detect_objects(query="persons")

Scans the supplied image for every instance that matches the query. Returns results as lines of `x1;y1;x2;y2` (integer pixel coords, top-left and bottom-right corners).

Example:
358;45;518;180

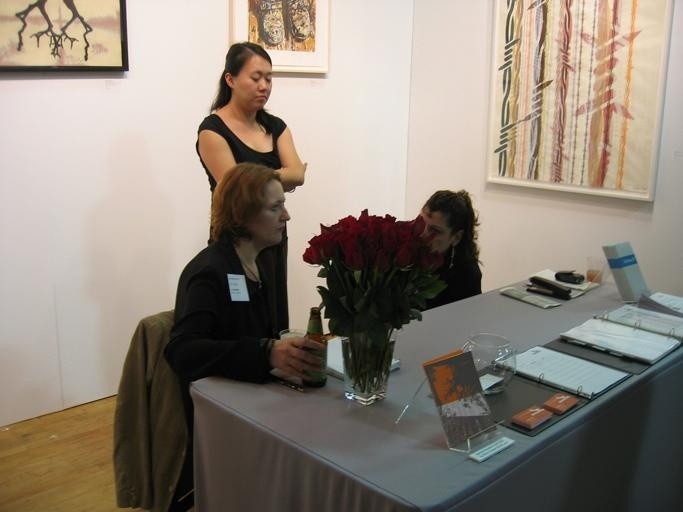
196;41;306;334
416;188;481;309
171;165;325;386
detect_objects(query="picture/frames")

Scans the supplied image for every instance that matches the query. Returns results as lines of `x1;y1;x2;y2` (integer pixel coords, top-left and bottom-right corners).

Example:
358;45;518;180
229;0;329;75
0;0;127;71
486;0;674;202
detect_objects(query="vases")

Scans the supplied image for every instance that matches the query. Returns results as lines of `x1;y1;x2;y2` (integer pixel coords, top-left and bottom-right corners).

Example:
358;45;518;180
342;337;396;405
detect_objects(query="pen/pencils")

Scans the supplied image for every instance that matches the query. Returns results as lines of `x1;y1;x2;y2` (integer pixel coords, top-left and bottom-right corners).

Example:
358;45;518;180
270;374;304;392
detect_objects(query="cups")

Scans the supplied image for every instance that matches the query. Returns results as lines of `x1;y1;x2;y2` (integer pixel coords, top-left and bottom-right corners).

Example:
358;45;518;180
585;255;606;283
278;329;302;339
461;332;516;395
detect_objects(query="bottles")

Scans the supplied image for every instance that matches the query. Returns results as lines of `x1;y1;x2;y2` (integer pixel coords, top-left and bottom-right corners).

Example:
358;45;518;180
301;307;328;388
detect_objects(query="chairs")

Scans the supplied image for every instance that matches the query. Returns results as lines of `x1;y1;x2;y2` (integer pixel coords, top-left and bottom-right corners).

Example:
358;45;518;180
114;310;225;512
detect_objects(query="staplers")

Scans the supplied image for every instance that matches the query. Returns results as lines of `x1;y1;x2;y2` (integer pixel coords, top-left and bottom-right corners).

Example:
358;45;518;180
525;276;572;301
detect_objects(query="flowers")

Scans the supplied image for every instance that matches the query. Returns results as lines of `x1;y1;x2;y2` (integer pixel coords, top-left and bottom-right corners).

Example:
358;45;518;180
302;209;448;390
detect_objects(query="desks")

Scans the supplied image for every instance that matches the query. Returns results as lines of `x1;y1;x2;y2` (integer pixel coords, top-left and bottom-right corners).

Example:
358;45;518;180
188;265;683;511
